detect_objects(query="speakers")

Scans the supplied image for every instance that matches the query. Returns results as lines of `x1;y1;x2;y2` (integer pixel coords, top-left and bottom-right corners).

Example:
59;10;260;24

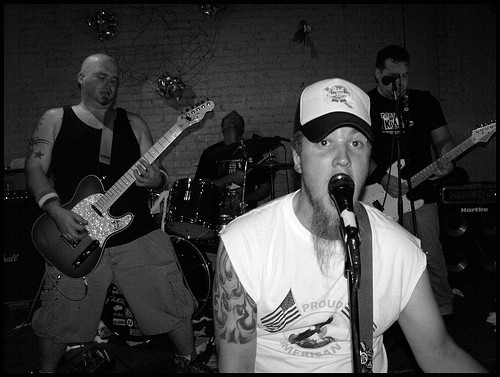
435;185;499;278
1;200;52;309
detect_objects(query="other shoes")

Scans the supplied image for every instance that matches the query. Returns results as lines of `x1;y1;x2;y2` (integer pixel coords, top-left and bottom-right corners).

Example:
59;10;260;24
169;354;211;374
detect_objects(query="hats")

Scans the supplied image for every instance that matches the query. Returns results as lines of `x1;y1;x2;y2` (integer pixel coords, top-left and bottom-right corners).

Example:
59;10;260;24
294;77;375;145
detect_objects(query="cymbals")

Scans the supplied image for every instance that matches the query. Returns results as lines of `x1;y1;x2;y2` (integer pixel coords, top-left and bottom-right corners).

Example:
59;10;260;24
227;135;281;156
249;161;294;170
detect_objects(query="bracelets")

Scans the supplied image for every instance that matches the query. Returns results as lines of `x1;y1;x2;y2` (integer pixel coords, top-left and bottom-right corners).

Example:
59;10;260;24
154;169;170;193
38;192;59;209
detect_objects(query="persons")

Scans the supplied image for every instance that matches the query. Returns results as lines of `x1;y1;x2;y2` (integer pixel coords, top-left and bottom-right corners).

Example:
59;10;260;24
364;43;457;374
212;77;492;374
193;110;277;211
24;53;217;377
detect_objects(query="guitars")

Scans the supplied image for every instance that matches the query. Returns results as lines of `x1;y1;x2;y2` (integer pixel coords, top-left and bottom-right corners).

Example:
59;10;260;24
30;97;216;279
358;119;498;224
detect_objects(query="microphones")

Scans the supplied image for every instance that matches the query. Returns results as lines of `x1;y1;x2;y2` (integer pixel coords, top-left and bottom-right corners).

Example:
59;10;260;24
382;74;399;86
329;172;362;270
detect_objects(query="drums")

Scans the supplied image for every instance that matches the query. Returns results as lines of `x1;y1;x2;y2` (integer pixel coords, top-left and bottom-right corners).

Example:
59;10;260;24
99;235;214;344
166;175;225;242
